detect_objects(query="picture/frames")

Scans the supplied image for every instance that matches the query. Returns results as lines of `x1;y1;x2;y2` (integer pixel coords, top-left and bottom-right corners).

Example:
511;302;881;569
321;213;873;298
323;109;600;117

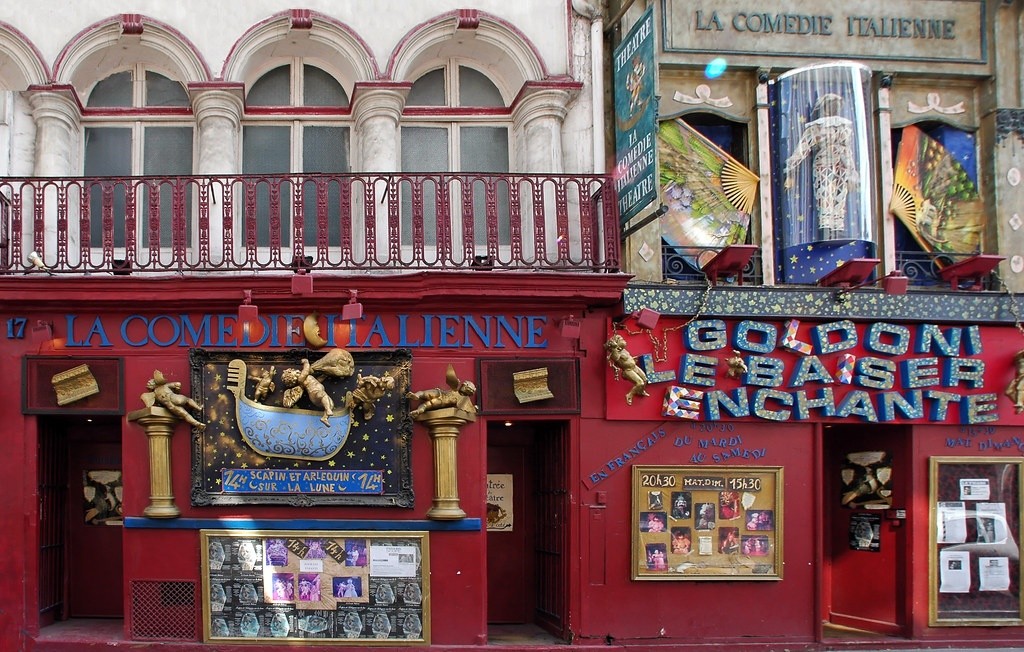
199;529;432;648
631;464;785;581
928;456;1024;626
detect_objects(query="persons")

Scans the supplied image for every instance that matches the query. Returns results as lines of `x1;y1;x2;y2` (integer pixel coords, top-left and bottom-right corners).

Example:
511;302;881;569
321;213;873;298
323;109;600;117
603;333;651;406
854;521;874;539
783;93;862;230
138;369;209;430
1004;349;1024;414
206;536;423;639
645;490;774;570
405;363;477;418
248;347;396;429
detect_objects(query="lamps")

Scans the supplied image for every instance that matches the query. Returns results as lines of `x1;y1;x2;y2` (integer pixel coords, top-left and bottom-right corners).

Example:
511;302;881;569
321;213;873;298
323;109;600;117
558;314;582;338
817;253;1007;304
32;319;53;342
471;255;494;271
613;308;661;330
27;251;55;277
238;256;363;321
112;259;132;276
701;243;759;287
